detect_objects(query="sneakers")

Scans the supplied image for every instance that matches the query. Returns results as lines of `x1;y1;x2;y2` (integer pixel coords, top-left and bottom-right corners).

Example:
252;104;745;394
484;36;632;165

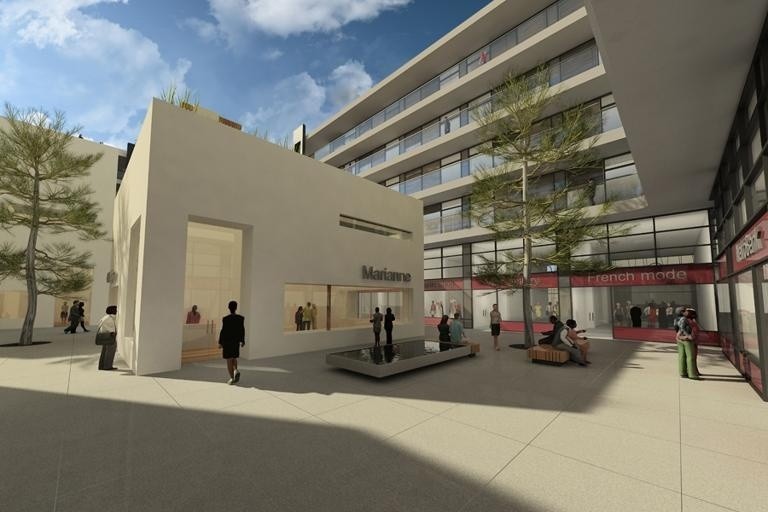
234;369;240;382
227;377;234;385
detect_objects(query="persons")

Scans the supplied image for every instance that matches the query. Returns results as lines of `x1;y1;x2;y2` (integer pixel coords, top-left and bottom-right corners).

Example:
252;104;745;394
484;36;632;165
613;300;706;381
429;300;436;317
294;302;318;331
383;307;395;344
489;304;502;351
449;313;470;343
437;314;451;342
218;301;246;384
98;306;119;371
60;300;91;334
370;307;383;346
186;305;200;324
533;300;592;367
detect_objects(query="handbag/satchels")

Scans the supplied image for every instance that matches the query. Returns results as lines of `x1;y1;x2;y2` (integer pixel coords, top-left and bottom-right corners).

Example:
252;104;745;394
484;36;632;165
95;332;115;345
